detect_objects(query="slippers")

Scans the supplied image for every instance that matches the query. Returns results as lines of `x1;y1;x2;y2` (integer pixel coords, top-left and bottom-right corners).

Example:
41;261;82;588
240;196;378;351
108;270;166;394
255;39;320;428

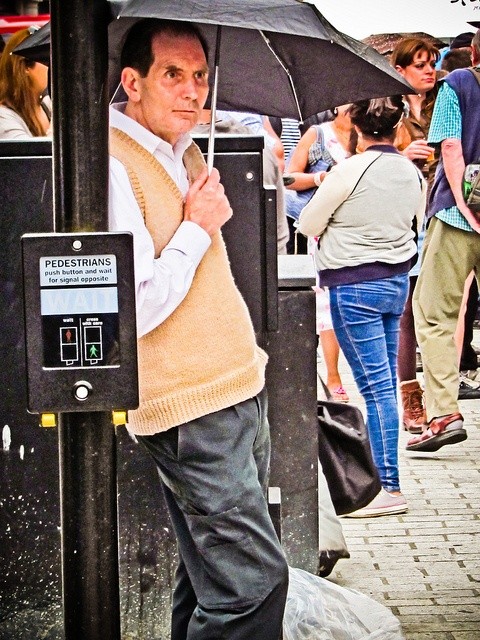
331;387;350;405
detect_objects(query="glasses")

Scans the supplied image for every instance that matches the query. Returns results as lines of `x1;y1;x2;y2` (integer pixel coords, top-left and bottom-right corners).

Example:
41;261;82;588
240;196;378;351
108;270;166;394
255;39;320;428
28;24;40;35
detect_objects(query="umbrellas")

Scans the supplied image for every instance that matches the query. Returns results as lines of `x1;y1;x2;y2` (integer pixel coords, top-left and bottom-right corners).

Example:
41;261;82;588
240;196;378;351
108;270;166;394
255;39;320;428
8;0;422;179
360;31;449;63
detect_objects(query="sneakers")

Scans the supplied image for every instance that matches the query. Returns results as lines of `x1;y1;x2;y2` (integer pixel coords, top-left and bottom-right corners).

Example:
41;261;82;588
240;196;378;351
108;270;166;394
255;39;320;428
341;486;407;518
458;382;479;399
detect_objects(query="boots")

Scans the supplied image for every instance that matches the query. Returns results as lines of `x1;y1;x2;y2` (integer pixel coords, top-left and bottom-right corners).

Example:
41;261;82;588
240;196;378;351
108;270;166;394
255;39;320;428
401;379;427;434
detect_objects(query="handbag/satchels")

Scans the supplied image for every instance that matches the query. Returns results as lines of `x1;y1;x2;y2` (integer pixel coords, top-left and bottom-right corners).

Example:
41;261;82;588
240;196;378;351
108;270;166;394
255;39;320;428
284;161;331;221
317;399;383;517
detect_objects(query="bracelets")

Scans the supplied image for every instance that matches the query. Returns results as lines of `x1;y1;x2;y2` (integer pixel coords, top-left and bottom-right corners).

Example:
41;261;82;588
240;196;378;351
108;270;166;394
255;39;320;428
313;171;325;186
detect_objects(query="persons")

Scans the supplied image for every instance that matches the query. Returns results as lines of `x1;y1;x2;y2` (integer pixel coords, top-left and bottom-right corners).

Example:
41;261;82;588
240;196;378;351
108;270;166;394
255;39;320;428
450;32;476;48
189;108;290;252
0;26;54;141
405;27;480;452
298;93;427;519
391;38;448;434
105;18;288;640
457;269;480;400
441;47;473;73
456;276;480;383
436;70;451;81
282;100;364;405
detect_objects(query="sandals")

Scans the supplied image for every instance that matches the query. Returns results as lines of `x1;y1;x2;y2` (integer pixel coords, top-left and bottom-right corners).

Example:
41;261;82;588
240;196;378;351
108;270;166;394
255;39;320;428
406;414;470;452
